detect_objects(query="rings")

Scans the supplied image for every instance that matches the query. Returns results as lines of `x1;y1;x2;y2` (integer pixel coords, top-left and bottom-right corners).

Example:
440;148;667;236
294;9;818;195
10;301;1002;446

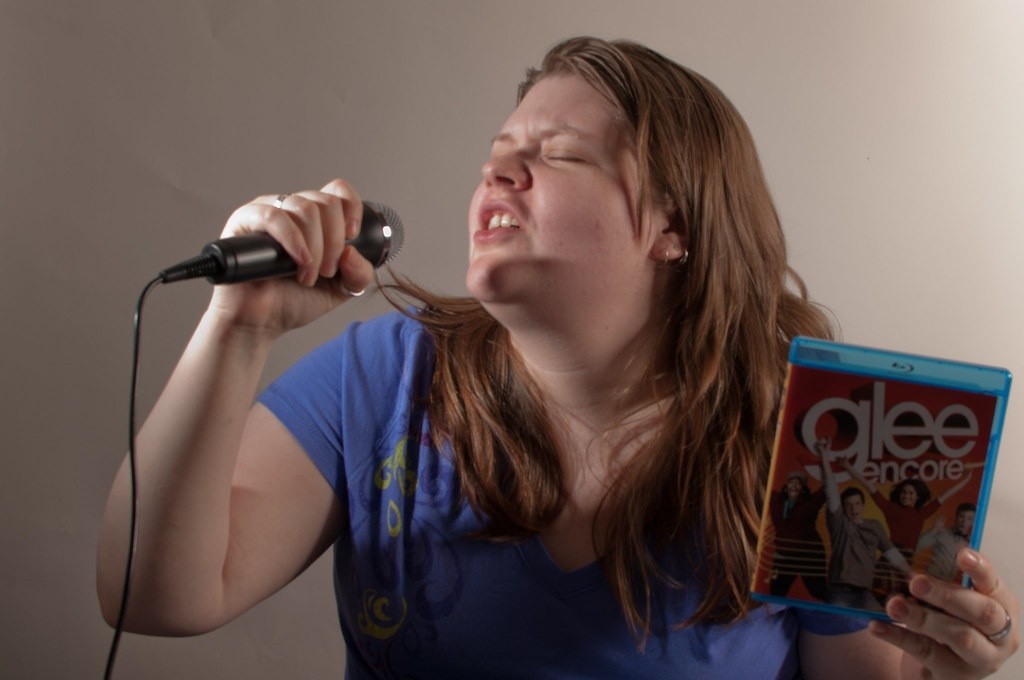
274;192;292;208
339;282;365;297
988;610;1013;640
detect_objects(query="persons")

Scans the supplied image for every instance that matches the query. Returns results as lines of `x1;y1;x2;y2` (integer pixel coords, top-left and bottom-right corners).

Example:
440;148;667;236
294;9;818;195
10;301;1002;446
835;453;974;608
814;435;914;612
766;475;831;601
915;500;976;584
94;35;1023;680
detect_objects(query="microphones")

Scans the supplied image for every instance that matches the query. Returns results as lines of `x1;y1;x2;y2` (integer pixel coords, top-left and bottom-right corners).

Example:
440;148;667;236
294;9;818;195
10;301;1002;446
159;201;406;289
